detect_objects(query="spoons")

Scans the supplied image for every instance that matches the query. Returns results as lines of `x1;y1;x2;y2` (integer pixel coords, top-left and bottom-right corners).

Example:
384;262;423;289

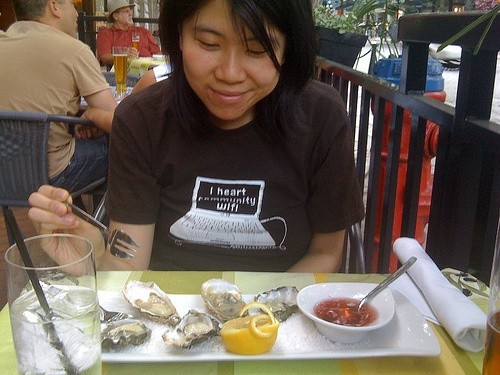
352;257;417;326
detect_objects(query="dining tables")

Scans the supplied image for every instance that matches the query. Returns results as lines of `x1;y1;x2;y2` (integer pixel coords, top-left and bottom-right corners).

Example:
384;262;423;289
0;272;489;375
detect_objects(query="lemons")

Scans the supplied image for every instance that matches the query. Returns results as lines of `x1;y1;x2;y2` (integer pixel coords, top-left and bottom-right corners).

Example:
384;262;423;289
220;302;280;354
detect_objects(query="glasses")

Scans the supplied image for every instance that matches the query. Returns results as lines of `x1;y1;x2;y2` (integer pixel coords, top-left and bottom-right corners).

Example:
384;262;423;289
116;8;133;14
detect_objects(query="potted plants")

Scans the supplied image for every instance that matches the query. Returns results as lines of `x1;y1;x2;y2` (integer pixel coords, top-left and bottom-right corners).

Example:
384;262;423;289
312;0;404;68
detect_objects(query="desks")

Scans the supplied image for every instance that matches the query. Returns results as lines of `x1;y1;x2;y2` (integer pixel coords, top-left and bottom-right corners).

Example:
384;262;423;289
78;71;137;111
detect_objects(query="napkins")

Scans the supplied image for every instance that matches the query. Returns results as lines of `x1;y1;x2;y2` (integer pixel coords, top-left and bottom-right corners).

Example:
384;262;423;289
388;237;487;351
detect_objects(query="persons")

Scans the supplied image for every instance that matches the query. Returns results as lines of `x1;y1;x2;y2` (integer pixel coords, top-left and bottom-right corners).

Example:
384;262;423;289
96;0;160;68
74;64;173;140
0;0;117;229
28;0;365;274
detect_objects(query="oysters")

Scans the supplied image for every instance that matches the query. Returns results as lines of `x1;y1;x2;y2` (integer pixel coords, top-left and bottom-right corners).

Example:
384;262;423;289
162;308;223;351
122;279;182;327
200;278;249;324
251;284;299;324
100;318;153;352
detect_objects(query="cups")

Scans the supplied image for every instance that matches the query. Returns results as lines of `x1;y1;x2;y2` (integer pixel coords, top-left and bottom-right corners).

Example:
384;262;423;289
131;32;140;59
4;234;103;375
112;45;129;104
480;220;500;374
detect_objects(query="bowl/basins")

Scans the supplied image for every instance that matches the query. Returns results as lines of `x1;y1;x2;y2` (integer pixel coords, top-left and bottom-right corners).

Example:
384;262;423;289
296;281;395;346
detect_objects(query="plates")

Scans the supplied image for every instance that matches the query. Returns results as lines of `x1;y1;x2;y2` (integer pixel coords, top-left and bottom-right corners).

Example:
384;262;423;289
98;289;441;362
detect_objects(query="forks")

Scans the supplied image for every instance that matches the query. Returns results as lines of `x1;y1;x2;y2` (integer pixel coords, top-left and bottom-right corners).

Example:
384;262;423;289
65;200;141;260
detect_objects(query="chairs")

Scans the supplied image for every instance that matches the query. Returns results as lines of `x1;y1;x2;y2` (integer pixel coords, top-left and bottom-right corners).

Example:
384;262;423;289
0;112;367;273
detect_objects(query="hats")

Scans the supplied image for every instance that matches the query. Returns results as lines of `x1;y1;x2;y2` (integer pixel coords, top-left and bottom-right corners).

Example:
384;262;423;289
107;0;135;23
